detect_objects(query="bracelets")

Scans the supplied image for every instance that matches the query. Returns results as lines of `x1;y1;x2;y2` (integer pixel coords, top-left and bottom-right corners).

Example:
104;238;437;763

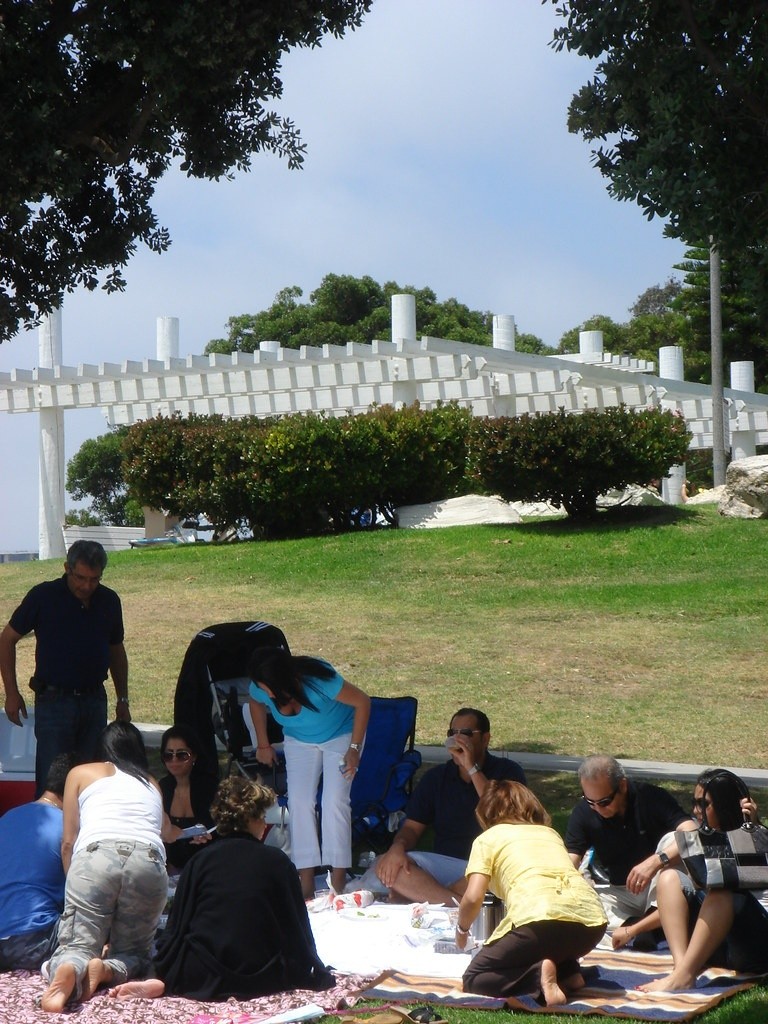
625;925;634;939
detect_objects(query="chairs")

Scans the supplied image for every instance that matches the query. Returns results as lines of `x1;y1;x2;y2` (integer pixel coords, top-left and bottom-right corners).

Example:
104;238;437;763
277;695;422;880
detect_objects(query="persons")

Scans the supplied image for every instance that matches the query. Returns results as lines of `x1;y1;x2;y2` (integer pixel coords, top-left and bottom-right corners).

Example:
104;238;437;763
1;619;768;1014
0;540;129;800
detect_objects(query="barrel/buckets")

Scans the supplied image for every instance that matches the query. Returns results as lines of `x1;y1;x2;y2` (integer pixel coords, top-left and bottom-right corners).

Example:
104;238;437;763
472;893;506;941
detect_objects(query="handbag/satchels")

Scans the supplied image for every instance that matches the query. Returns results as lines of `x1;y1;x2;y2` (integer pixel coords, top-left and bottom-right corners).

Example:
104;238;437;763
674;774;768;891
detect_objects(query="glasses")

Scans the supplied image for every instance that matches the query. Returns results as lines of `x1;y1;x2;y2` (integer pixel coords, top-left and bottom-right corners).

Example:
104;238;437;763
447;727;482;737
68;567;102;583
692;797;713;808
584;784;618;807
162;751;194;762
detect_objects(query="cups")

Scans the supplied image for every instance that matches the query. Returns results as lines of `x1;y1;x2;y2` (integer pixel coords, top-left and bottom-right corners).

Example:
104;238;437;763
315;888;333;912
444;909;459;932
445;735;461;751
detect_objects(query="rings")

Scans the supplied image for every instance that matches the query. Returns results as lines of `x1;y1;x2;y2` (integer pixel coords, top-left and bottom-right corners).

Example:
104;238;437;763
637;881;642;886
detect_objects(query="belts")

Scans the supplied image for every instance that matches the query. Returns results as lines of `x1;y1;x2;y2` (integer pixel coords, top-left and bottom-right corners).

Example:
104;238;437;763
45;685;83;695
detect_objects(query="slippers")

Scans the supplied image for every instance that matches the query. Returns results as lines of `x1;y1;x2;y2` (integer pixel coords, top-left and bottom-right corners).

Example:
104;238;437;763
341;1013;404;1024
389;1003;449;1024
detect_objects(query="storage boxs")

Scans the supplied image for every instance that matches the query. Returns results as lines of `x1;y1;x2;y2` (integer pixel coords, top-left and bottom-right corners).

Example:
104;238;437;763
0;707;39;816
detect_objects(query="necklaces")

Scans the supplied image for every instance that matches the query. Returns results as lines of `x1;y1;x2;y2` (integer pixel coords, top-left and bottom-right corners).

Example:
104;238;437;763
41;796;63;811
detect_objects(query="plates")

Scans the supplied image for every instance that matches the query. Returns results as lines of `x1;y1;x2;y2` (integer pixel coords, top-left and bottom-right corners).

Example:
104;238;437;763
338;908;390;922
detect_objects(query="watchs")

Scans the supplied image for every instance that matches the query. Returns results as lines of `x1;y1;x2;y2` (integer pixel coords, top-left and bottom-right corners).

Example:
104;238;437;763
467;763;481;775
348;743;362;753
117;698;129;705
657;850;670;869
456;923;469;935
